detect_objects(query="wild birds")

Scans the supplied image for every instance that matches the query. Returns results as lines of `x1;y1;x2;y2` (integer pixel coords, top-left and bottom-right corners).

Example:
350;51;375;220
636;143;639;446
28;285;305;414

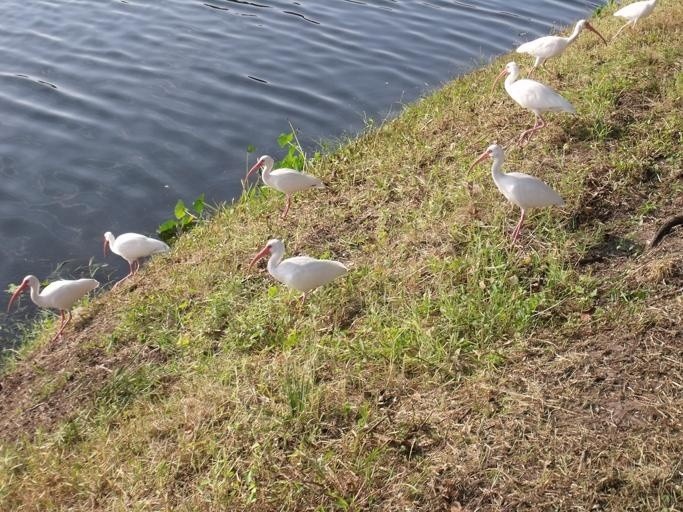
612;0;658;39
467;144;567;253
492;61;577;146
516;19;607;79
249;239;350;308
246;155;326;218
103;232;171;291
7;275;100;342
